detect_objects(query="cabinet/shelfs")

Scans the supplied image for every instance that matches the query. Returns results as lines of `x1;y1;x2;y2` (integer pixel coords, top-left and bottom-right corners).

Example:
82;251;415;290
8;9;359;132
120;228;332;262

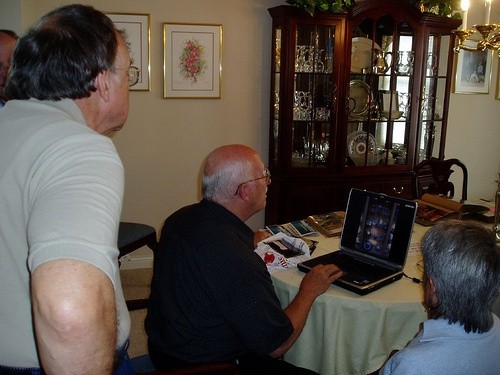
265;0;462;229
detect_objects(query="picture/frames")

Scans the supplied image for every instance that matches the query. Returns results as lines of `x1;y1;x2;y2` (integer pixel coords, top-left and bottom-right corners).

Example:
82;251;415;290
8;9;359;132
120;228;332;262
161;22;222;99
100;12;151;92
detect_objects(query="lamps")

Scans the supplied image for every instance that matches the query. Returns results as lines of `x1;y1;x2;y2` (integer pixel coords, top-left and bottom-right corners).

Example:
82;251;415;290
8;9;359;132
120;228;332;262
453;0;500;57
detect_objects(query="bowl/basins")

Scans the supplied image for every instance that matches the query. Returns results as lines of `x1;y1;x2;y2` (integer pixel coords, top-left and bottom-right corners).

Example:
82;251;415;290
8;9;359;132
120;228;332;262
378;149;405;164
382;111;404;119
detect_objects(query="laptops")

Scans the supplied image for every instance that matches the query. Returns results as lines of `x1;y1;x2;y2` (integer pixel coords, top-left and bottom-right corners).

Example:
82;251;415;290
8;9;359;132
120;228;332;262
296;187;418;296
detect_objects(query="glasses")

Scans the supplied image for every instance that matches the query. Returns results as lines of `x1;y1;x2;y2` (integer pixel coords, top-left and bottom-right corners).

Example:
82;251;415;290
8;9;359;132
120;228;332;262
417;259;425;272
234;168;271;195
109;65;140;86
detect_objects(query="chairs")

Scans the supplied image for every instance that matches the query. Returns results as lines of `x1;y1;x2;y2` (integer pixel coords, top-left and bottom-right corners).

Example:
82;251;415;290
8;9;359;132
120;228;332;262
412;157;468;201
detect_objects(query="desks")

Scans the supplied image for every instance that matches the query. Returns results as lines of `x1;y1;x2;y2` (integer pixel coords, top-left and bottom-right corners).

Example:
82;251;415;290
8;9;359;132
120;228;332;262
269;201;500;375
453;37;492;95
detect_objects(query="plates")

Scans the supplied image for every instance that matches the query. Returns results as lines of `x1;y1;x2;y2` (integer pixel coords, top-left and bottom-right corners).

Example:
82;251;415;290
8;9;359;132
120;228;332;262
349;80;373;116
350;37;381;73
348;131;377;163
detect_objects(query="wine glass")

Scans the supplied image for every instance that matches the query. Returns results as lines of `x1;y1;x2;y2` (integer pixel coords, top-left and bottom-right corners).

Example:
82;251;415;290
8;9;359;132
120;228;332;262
295;44;325;72
274;90;330;120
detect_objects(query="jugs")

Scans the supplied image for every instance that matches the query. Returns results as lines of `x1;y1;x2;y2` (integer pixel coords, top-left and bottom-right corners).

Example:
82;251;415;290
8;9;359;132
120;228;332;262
370;48;438;76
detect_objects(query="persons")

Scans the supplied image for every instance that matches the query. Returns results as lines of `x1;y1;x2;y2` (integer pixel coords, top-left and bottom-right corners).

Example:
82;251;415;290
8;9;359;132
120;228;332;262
463;59;484;83
378;219;500;375
0;3;141;375
143;145;344;375
